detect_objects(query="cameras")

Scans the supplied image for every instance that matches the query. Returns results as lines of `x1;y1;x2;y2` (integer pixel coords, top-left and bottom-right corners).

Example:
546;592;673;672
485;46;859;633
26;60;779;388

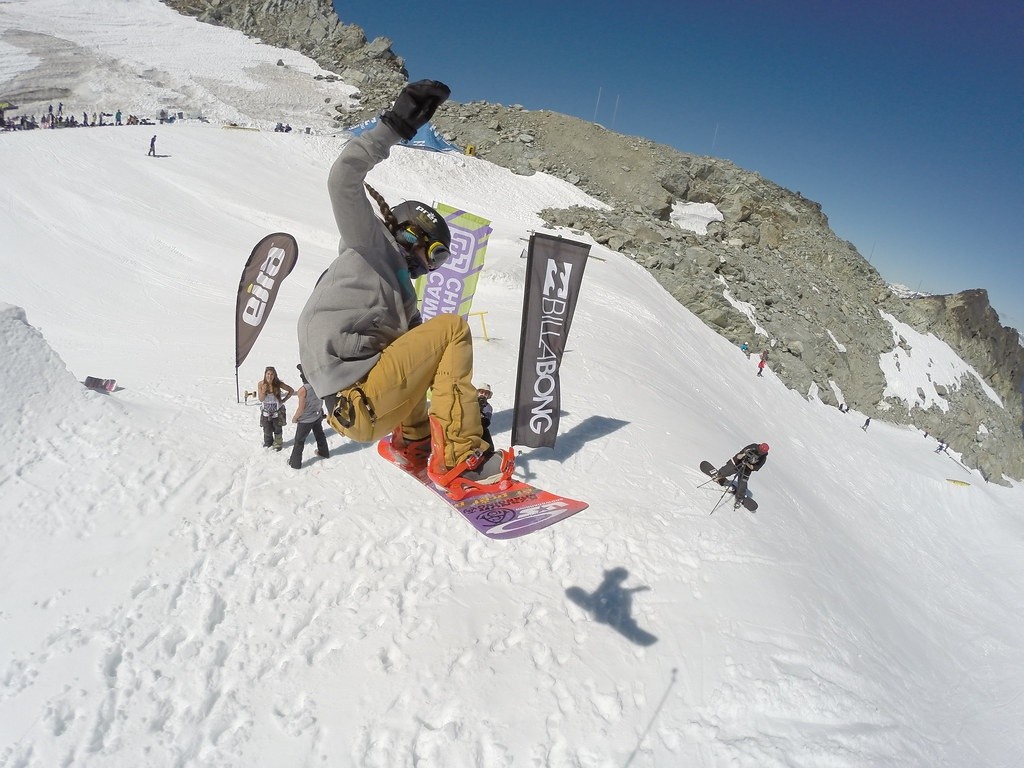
477;394;488;407
744;455;751;461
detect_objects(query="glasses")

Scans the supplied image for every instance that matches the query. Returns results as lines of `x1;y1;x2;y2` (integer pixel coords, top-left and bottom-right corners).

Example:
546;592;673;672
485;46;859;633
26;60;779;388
266;366;275;370
410;225;451;271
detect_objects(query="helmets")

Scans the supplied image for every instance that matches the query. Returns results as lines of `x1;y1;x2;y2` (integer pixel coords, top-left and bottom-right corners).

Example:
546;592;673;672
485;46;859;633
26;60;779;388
758;442;769;454
389;199;451;271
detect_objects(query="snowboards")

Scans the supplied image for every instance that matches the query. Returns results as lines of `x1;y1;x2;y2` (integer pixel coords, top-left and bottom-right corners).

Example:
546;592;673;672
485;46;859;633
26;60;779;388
699;459;759;512
82;375;118;392
376;434;590;542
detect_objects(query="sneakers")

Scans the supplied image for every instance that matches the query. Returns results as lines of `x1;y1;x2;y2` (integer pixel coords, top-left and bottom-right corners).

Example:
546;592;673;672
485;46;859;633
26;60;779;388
287;459;301;469
394;436;431;464
314;448;329;457
433;450;515;493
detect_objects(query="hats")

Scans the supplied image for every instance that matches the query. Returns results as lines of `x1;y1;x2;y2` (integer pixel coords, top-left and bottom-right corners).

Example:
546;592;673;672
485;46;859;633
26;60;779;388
745;342;748;345
297;364;303;372
477;382;493;400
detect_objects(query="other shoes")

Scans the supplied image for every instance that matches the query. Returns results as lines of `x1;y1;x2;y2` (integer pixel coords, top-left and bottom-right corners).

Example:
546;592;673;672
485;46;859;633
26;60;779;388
734;500;743;508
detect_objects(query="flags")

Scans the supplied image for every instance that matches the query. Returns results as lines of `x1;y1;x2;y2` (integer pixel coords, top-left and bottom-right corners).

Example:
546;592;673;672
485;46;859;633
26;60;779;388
510;233;592;448
414;202;493;324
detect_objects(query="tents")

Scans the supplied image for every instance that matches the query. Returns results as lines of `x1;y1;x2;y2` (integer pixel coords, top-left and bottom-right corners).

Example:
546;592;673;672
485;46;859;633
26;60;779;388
346;114;453;153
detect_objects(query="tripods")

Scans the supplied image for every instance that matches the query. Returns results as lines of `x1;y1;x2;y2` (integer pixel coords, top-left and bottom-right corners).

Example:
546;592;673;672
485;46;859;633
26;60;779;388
697;461;748;516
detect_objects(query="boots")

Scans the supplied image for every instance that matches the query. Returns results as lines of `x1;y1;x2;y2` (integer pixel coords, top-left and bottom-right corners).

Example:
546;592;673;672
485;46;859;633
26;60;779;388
263;432;274;449
274;434;283;452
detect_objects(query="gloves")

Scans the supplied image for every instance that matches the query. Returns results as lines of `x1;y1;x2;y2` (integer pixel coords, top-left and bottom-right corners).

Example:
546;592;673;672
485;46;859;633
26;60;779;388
382;78;451;140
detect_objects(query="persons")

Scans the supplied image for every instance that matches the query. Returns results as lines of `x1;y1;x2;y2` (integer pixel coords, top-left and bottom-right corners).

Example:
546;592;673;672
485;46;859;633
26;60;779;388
198;116;211;123
296;78;516;492
756;349;770;378
861;417;872;432
275;122;292;133
148;135;158;157
935;442;950;453
475;384;495;458
257;367;294;452
712;443;769;512
985;473;992;483
0;102;176;131
839;403;851;414
287;363;330;469
924;428;931;438
741;341;751;360
